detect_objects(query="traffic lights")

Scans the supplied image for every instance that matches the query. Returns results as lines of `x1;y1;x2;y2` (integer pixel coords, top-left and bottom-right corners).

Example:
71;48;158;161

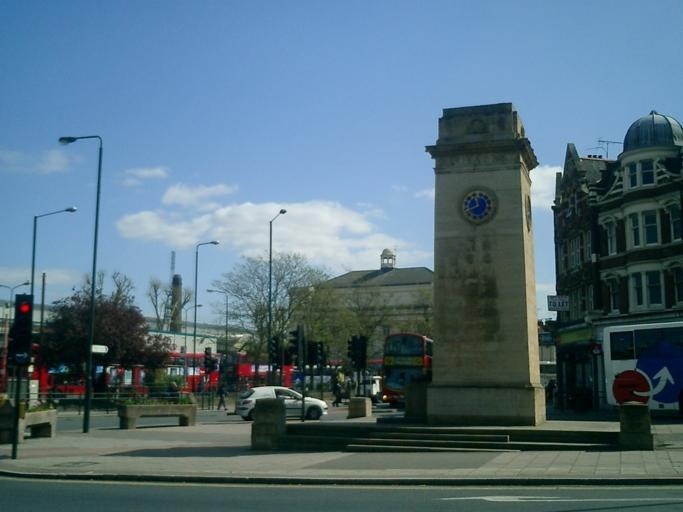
346;334;359;361
268;334;283;369
290;322;306;367
11;292;33;367
313;341;324;363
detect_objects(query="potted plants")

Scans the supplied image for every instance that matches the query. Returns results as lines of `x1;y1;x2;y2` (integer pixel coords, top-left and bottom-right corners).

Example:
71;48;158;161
0;399;57;443
118;396;198;429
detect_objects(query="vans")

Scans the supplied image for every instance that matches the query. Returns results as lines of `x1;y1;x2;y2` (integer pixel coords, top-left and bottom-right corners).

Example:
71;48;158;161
353;375;383;403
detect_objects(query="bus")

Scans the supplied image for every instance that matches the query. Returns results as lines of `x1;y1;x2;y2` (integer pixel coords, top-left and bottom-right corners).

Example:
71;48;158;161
288;363;363;391
2;347;297;395
600;319;682;420
539;360;557;393
325;359;383;377
378;331;433;410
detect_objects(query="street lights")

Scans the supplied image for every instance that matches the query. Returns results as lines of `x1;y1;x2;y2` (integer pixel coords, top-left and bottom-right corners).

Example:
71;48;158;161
29;203;81;301
189;238;224;393
54;132;103;432
205;286;230;363
182;304;205;389
1;277;32;383
266;205;287;384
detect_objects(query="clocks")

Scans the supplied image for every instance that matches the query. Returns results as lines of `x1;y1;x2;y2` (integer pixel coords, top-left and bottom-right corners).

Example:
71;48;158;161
525;195;531;231
459;186;498;226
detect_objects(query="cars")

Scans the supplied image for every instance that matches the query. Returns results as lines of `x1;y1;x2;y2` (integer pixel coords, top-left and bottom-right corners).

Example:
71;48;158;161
232;383;330;421
